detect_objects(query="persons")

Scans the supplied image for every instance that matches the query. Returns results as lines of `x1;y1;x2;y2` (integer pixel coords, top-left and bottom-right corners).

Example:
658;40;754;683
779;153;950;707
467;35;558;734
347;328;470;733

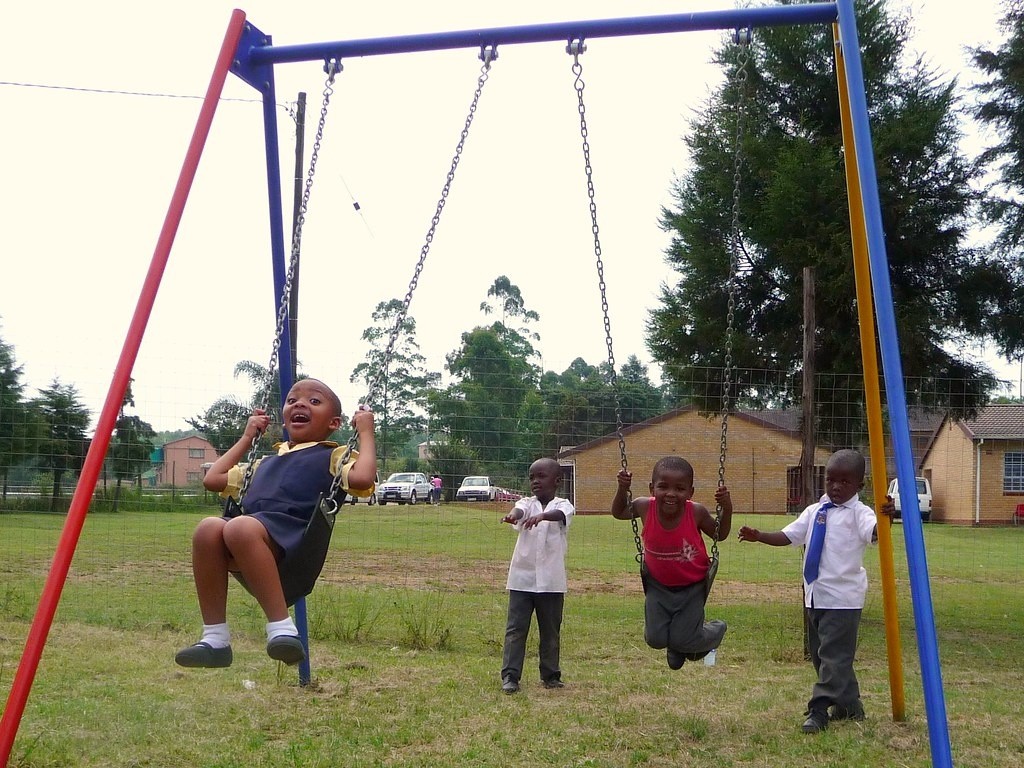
430;475;441;504
611;455;733;671
738;449;896;734
499;458;575;695
175;378;377;668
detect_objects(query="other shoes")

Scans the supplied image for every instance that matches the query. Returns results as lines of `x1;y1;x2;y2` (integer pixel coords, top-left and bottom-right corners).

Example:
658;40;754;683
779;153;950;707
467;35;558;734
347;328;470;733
267;635;305;666
175;641;233;668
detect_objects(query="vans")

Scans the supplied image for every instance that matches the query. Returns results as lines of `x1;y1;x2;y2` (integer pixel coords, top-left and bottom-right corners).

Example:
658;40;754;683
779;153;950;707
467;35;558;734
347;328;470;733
888;476;933;521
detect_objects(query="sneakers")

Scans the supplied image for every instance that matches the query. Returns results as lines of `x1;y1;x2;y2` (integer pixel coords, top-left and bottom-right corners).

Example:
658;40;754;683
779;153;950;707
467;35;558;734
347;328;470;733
686;649;713;661
667;646;686;670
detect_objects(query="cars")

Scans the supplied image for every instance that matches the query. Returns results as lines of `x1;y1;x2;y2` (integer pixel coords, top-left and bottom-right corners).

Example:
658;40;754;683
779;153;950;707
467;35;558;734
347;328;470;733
494;487;522;502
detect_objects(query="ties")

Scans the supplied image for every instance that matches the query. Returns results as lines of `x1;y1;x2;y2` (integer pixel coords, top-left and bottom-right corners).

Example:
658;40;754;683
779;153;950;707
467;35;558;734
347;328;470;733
804;502;834;585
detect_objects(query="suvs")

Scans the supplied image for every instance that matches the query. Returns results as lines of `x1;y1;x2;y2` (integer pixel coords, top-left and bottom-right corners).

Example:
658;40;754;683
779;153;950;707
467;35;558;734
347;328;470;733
455;476;495;502
341;471;380;506
376;472;435;505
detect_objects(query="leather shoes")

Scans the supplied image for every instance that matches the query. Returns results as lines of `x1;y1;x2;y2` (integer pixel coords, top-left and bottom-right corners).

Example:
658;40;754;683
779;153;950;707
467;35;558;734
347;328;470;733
801;711;828;731
503;674;519;694
542;679;564;689
828;707;865;720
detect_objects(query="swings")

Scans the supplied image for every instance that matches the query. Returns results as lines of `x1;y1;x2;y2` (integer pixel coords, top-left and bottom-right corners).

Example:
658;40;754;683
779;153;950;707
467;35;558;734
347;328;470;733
218;51;490;608
572;43;751;601
